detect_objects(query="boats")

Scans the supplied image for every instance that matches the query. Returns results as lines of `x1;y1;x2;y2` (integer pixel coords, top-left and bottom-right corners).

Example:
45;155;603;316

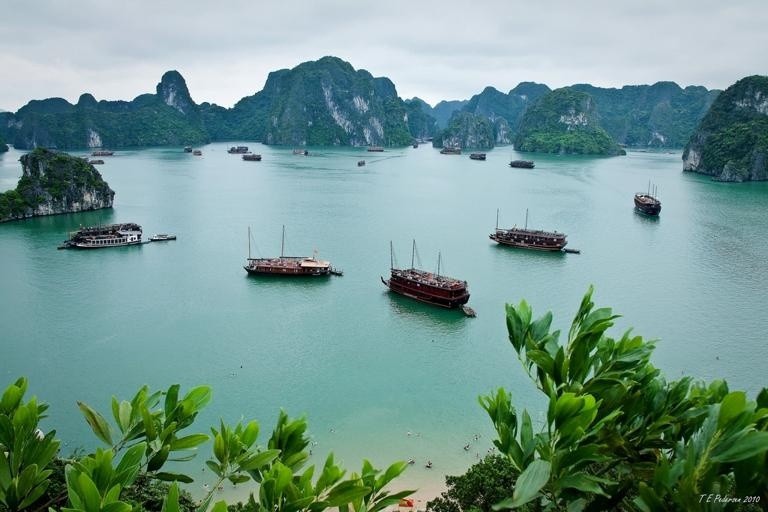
292;143;486;166
184;146;202;156
57;215;176;249
89;150;115;164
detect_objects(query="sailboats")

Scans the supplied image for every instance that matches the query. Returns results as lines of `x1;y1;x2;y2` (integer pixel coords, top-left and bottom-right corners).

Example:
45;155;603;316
488;208;580;254
243;225;343;277
381;239;478;318
226;143;261;161
507;153;535;168
633;179;661;216
